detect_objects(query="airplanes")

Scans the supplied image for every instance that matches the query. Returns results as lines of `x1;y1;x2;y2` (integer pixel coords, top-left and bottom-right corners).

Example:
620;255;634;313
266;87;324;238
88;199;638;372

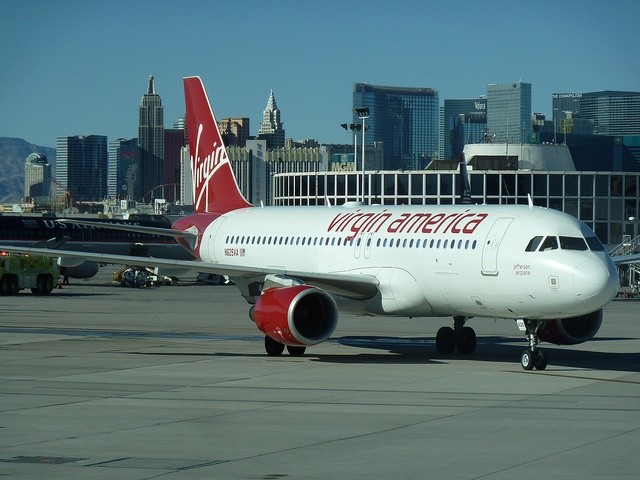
0;76;620;370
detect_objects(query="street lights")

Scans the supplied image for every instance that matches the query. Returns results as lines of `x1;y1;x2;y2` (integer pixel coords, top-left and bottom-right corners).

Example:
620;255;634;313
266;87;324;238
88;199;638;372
355;107;369;202
339;123;368;171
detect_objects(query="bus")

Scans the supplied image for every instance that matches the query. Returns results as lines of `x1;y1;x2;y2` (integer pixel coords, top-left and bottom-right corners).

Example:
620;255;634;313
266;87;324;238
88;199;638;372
1;251;59;297
